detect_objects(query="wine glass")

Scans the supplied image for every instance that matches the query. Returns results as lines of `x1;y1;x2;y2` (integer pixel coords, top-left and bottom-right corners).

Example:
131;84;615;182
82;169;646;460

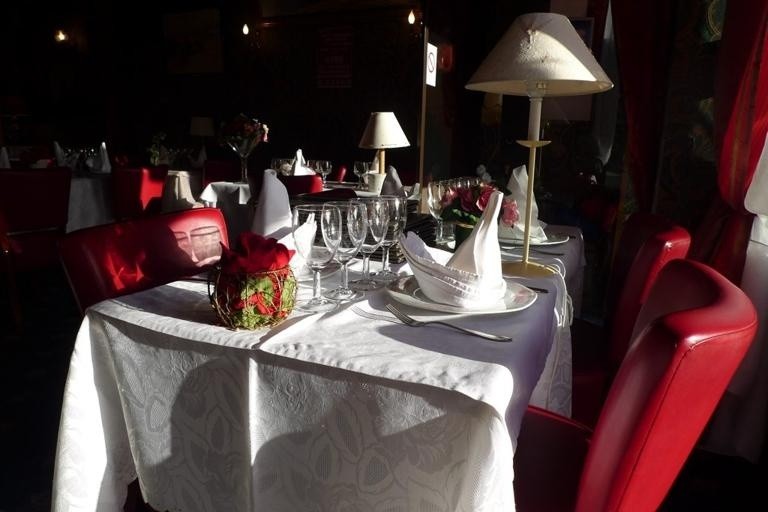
172;226;222;262
269;156;374;189
292;194;408;314
426;175;480;244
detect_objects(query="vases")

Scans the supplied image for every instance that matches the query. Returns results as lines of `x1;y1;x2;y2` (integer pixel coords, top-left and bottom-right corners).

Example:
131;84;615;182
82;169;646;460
454;221;480;251
211;269;297;331
223;134;261;182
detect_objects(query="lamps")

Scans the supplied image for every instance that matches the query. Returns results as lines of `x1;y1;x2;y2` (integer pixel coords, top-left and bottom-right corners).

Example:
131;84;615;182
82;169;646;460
464;12;615;279
357;111;412;172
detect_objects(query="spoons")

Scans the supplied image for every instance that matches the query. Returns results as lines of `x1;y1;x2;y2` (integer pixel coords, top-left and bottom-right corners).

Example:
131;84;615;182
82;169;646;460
385;302;513;342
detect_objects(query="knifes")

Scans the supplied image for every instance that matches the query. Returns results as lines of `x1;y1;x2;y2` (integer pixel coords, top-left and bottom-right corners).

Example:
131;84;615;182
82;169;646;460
499;246;566;257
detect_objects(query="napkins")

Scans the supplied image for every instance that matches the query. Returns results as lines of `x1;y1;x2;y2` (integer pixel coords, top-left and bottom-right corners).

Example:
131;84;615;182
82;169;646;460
380;166;406;198
400;189;504;310
494;166;548;244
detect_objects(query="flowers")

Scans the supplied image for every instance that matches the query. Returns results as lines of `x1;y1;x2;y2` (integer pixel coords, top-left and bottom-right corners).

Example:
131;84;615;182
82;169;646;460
438;181;519;228
220;111;271;143
208;229;297;330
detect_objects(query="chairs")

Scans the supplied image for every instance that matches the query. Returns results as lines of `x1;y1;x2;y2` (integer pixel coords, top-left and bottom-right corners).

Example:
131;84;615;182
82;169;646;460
572;226;691;421
513;259;758;510
1;147;360;318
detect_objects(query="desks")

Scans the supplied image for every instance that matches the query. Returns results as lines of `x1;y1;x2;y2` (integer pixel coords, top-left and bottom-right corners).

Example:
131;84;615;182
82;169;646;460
434;226;586;318
88;254;556;511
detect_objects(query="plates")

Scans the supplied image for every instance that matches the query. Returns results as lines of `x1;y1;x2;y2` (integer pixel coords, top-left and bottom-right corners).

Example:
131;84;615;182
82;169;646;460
497;229;570;245
386;277;537;317
